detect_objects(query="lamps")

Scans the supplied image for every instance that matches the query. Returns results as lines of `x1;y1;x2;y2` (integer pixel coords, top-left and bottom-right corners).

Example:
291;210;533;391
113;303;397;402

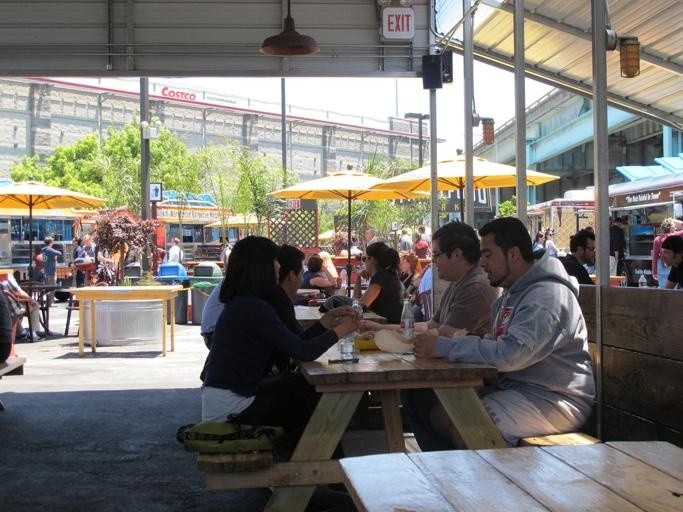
471;112;494;145
258;0;321;57
605;28;639;77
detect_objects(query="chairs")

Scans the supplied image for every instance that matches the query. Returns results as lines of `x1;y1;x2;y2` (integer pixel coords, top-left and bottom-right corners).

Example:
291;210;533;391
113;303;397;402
0;278;108;342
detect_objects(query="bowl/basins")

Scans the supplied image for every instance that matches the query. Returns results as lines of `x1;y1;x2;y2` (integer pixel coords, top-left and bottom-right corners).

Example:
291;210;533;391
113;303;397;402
587;275;625;286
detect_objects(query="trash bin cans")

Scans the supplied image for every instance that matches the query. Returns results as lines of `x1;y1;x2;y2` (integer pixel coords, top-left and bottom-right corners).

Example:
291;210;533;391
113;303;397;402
191;261;224;324
156;262;191;324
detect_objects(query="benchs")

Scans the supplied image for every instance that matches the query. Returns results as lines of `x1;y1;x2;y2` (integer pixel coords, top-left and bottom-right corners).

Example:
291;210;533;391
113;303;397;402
194;421;280;492
0;355;26;412
517;430;602;446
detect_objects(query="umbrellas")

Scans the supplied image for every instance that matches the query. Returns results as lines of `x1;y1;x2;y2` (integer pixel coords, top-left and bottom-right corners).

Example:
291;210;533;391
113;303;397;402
1;175;108;295
203;210;291;238
379;147;564;221
267;163;430;297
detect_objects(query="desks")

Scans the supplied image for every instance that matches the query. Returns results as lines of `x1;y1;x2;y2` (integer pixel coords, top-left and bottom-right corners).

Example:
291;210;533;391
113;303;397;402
250;302;509;511
337;440;683;512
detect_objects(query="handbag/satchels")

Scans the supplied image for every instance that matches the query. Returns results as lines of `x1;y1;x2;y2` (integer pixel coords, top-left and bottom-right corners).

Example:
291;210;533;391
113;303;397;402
319;295;370;313
34;253;45;263
174;413;284;455
617;259;640;286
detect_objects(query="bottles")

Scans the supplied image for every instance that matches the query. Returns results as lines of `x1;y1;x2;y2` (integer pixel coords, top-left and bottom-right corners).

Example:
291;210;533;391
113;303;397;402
620;271;627;287
351;300;364;319
339;334;355;364
637;275;647;288
400;298;415;338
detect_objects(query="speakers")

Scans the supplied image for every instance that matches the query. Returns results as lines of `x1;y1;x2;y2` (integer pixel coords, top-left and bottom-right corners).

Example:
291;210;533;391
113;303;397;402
441;50;454;85
423;53;442;87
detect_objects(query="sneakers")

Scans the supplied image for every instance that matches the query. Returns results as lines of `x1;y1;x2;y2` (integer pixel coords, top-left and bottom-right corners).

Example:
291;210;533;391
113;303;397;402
327;482;349;496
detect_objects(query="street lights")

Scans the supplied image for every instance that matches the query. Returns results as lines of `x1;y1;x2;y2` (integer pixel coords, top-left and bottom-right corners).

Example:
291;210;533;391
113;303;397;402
404;112;429;168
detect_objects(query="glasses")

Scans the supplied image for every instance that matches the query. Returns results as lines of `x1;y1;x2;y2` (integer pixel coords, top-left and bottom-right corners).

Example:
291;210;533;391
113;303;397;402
362;255;370;263
432;250;447;258
584;247;596;252
548;234;554;236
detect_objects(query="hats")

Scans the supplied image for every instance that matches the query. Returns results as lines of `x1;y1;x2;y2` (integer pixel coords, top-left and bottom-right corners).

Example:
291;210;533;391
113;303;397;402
661;235;683;251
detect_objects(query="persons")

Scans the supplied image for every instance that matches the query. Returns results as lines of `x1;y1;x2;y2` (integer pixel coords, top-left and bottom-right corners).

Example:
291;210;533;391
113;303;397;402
202;234;363;493
0;237;183;363
531;216;683;290
353;221;497;432
412;217;596;445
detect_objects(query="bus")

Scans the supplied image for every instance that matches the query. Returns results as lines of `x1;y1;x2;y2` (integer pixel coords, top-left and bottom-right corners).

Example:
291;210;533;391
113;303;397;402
0;209;104;270
